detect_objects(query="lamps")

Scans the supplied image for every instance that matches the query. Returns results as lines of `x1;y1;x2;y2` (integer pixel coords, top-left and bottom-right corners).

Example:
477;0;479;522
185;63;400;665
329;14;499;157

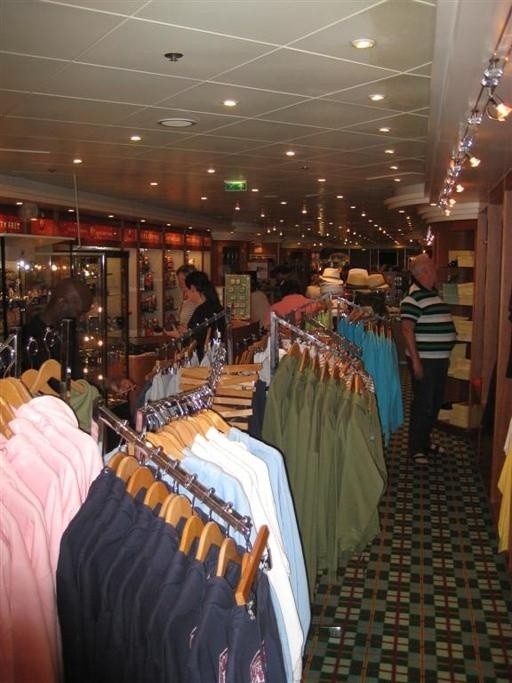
436;43;511;219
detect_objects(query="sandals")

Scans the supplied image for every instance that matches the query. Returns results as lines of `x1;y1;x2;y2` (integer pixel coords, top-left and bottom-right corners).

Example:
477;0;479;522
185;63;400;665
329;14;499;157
426;443;447;456
412;452;429;467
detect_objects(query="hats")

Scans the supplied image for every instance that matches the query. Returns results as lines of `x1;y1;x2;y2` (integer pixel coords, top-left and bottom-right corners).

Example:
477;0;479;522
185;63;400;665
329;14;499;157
319;267;390;293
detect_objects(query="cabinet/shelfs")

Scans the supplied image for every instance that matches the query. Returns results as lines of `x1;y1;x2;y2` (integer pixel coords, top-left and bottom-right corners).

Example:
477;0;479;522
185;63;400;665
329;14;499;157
0;198;248;449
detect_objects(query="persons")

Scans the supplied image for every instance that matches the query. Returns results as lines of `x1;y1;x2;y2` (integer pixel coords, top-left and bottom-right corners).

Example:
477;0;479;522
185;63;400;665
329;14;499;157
174;263;198;329
250;280;271;320
163;270;227;364
19;277;93;394
399;252;466;465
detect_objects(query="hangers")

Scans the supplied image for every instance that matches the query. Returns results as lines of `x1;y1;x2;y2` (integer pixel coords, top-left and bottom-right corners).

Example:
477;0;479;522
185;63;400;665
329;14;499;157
2;324;270;618
274;294;400;400
